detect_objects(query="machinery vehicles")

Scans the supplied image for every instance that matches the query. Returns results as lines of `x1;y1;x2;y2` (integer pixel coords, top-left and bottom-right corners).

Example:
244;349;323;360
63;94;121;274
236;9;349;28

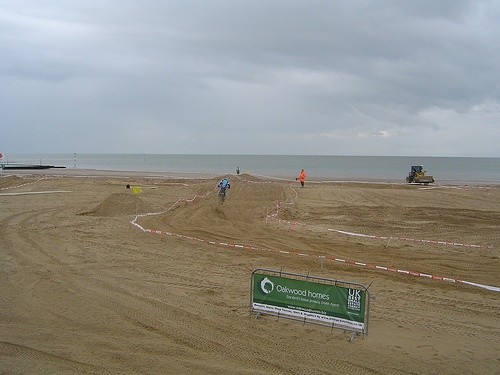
406;165;435;183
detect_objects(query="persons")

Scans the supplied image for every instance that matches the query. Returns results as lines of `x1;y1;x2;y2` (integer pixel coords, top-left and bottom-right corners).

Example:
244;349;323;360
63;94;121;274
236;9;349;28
216;177;231;203
297;168;306;188
235;166;241;175
